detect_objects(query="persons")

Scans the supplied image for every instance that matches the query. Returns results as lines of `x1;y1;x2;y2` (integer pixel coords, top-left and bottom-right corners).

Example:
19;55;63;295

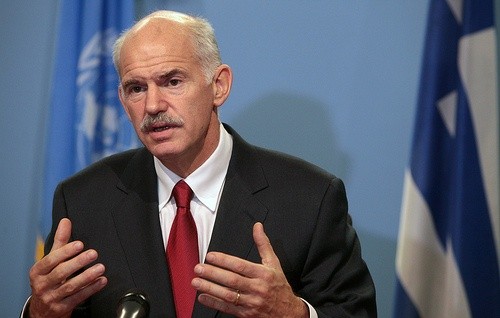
20;10;378;318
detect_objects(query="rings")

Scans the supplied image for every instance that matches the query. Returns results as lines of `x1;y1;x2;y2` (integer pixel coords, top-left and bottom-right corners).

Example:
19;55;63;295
235;290;240;306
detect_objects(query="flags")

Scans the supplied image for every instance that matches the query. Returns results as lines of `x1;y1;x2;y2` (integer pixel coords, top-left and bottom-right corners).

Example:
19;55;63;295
34;0;145;262
391;0;499;318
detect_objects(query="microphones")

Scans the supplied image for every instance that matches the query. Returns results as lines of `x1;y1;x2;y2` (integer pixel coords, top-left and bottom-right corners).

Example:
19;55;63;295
115;288;151;318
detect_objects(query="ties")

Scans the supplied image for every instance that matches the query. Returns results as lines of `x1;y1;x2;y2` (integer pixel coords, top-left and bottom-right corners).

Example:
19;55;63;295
165;180;199;318
